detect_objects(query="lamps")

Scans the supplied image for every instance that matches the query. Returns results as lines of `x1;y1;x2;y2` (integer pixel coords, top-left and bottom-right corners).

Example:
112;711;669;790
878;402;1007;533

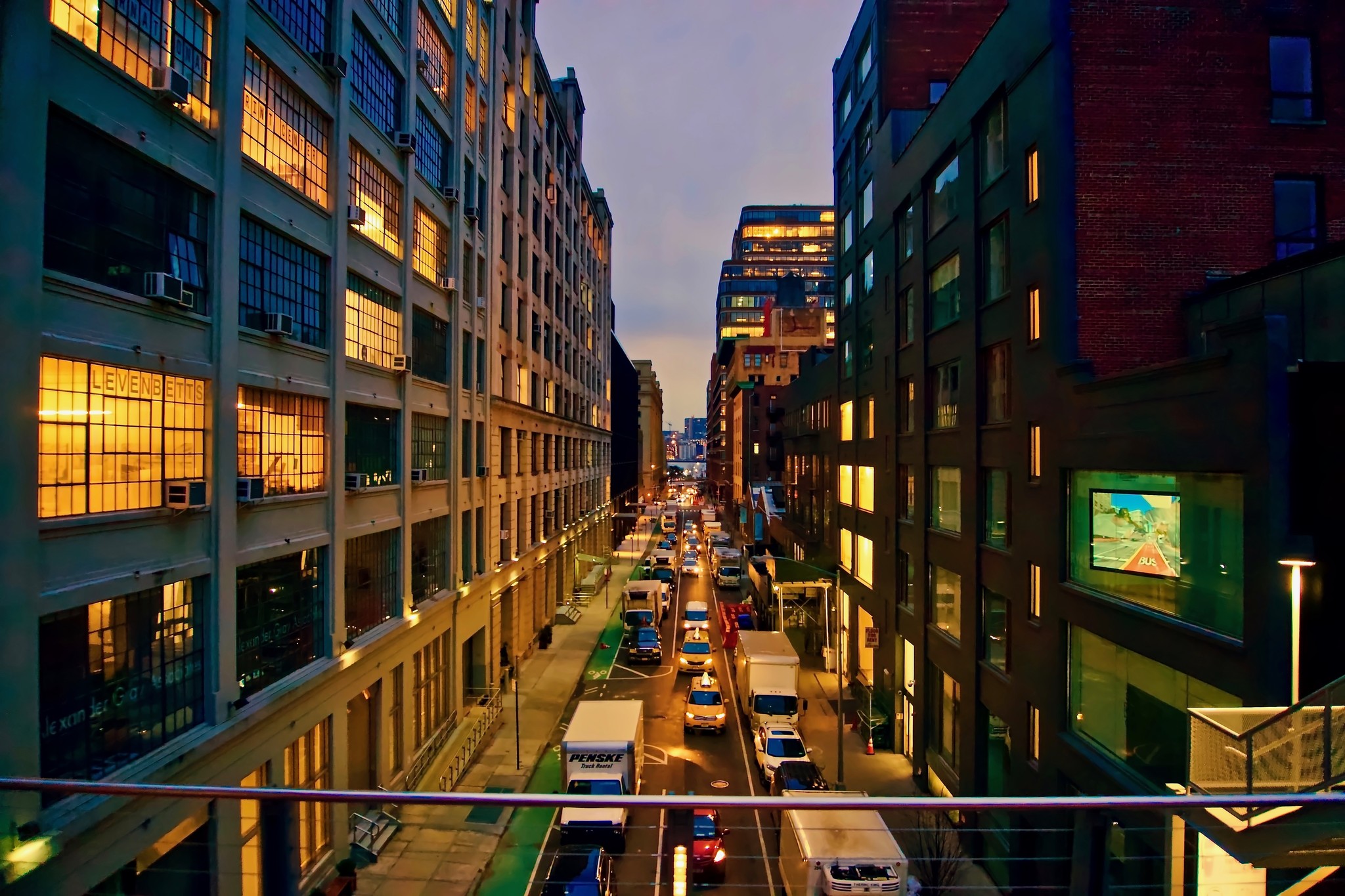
462;552;519;584
409;605;419;613
232;698;250;710
342;638;354;650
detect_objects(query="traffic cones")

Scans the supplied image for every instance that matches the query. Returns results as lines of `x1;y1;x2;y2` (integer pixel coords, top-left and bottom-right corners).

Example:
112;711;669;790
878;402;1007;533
866;736;875;755
599;643;611;649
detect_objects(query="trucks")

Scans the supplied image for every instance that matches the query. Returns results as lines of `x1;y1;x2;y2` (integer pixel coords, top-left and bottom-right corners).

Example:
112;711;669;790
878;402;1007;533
553;700;645;854
778;789;908;896
619;580;663;638
700;510;742;587
661;501;678;534
717;601;808;737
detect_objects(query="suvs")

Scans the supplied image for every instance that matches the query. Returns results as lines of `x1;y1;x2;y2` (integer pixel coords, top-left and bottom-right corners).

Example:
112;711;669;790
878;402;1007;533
754;721;830;832
660;583;670;619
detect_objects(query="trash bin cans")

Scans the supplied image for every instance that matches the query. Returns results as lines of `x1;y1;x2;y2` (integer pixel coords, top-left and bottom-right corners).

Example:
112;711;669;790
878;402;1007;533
861;707;889;747
538;624;553;650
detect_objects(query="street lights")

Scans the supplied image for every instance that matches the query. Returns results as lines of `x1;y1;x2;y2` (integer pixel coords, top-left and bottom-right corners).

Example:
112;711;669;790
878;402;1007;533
752;555;846;792
626;535;633;567
604;568;608;609
644;519;646;541
512;679;519;771
700;478;719;504
654;502;660;515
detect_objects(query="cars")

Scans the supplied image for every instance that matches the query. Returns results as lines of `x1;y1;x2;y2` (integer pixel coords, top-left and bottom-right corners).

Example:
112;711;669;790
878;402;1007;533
677;601;730;736
668;478;701;502
538;845;616;896
642;533;677;619
663;803;730;883
624;627;662;666
681;520;700;579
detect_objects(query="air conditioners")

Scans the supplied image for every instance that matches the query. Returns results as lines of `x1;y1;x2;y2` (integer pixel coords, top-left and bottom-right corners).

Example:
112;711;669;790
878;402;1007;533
320;51;347;78
345;473;367;490
165;481;206;508
348;206;365;225
145;272;193;308
417;48;430;69
265;312;294;335
465;206;480;220
412;469;427;482
391;355;412;371
443;186;460;203
236;476;265;502
442;278;458;292
476;466;489;477
151;66;190;105
394;131;417;153
